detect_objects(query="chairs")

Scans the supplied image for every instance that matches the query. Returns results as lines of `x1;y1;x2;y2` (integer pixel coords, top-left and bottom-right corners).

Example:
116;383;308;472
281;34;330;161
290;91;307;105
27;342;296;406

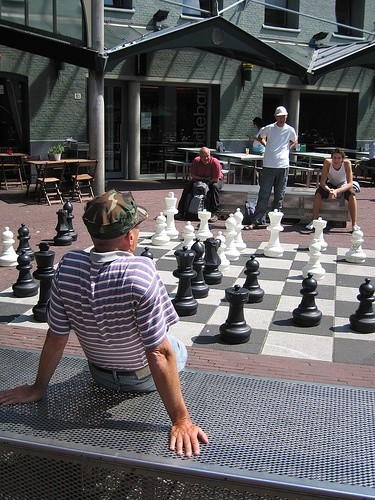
0;155;98;206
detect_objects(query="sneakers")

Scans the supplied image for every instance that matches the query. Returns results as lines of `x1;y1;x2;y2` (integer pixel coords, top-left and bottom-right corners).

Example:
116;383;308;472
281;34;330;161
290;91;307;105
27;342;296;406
305;220;314;229
352;225;360;231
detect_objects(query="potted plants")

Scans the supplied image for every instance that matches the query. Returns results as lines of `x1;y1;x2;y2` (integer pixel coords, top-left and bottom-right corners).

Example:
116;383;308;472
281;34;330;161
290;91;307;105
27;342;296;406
49;145;64;161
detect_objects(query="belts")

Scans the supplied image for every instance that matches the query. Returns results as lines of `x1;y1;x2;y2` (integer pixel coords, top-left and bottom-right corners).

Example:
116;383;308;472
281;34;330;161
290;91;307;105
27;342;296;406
91;363;136;376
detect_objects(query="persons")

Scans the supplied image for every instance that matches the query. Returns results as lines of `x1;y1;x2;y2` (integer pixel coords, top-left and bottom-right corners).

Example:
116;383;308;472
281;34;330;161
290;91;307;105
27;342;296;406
306;147;361;231
0;190;208;457
369;139;375;167
244;106;298;230
245;116;266;167
175;147;223;223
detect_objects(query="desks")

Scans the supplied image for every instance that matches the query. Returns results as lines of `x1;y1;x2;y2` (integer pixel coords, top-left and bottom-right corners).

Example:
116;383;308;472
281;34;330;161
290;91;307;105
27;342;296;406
315;146;354;155
213;152;264;185
293;151;348;185
0;153;25;188
178;146;230;163
26;159;98;203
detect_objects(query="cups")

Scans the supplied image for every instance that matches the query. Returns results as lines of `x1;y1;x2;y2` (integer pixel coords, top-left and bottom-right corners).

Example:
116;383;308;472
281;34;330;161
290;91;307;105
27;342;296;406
246;148;249;154
296;144;300;151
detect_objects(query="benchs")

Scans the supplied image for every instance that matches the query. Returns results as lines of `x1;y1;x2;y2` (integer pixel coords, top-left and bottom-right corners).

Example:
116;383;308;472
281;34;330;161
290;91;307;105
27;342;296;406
165;160;375;186
219;183;350;224
0;345;375;500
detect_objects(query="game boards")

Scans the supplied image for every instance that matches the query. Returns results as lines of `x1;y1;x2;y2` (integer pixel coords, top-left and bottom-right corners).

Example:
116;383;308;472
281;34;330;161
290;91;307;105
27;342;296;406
0;230;375;367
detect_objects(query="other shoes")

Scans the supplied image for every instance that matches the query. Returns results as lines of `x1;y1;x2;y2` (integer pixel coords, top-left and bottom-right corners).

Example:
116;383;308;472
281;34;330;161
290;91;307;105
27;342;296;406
208;213;218;222
244;222;260;230
174;210;185;220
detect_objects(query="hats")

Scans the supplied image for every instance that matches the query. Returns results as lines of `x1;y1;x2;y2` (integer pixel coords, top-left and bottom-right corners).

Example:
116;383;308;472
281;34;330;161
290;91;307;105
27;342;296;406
82;190;149;240
275;106;288;116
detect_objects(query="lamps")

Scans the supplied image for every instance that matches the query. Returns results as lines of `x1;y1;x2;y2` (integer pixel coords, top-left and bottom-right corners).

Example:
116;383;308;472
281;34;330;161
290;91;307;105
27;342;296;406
312;32;329;41
153;10;170;21
